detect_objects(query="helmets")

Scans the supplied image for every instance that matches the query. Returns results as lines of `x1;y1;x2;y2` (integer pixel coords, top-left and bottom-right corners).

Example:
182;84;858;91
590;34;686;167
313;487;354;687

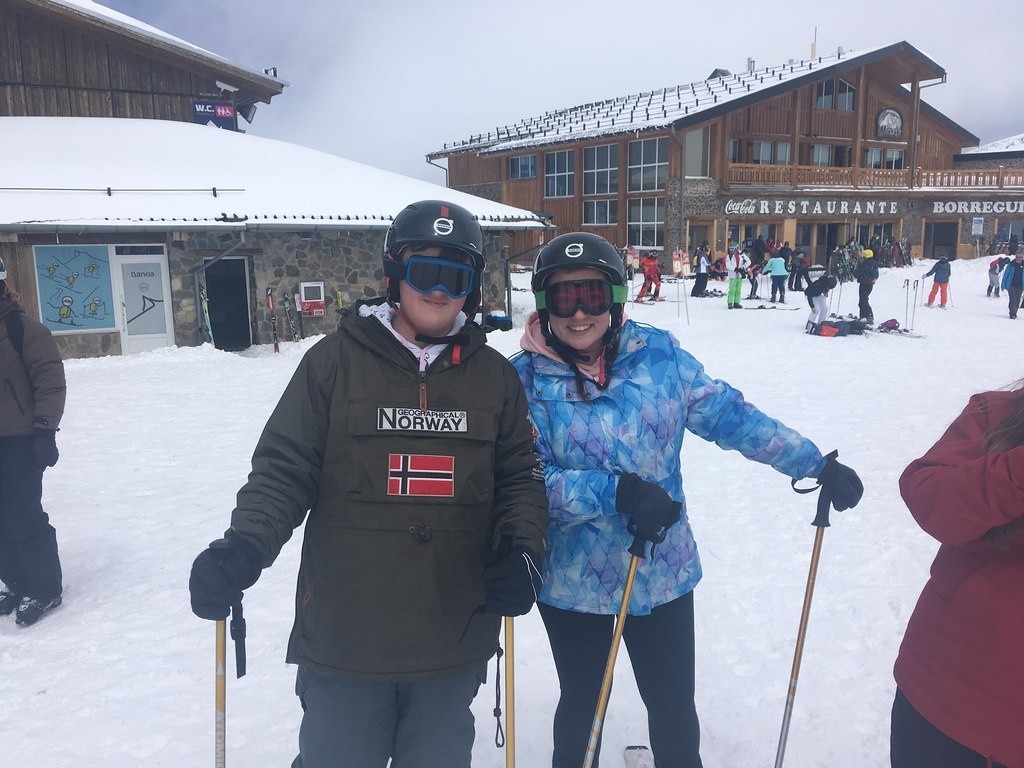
862;250;874;259
383;201;486;279
530;232;626;295
729;242;739;251
939;253;948;262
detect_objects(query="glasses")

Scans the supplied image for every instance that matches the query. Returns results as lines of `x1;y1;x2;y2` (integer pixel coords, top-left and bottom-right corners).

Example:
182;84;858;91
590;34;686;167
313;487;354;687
544;279;615;316
404;255;474;299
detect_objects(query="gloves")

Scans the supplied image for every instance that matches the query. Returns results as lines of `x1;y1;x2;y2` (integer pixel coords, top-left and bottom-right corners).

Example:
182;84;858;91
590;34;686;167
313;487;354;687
481;530;543;617
817;457;863;510
615;472;676;543
647;275;651;280
922;274;927;279
188;540;254;621
34;427;59;468
734;268;740;272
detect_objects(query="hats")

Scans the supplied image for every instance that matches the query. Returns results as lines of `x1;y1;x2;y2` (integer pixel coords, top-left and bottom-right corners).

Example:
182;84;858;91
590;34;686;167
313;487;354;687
827;276;837;289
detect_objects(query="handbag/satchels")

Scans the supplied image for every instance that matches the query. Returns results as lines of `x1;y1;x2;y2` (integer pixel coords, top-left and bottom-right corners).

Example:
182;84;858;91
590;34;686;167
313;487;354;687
812;320;850;338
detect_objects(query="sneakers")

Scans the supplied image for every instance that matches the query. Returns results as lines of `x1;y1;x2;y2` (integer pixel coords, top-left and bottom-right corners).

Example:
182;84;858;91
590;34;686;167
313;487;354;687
0;582;19;615
14;593;63;625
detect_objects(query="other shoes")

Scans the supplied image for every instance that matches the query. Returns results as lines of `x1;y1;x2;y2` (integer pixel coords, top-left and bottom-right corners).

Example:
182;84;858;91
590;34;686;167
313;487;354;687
652;297;661;301
635;297;642;302
925;302;931;307
938;303;946;309
859;316;871;323
728;302;743;308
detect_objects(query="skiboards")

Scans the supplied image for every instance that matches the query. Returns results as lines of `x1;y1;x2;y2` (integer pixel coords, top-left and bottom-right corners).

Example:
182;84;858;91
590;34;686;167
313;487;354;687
624;745;655;768
627;298;683;305
838;236;912;282
924;303;947;310
768;300;788;304
826;313;859;321
897;328;928;339
975;233;1007;258
742;297;766;300
199;283;343;353
745;306;800;310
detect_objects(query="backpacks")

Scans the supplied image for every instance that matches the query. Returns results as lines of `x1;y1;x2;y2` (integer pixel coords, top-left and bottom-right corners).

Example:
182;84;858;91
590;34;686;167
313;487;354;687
877;319;899;331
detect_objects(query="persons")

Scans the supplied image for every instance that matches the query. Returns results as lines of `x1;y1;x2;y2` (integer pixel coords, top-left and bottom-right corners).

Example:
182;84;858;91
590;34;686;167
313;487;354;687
987;241;1024;318
889;384;1024;768
804;276;838;334
690;234;812;309
189;199;548;768
613;243;636;279
507;231;863;768
0;258;66;626
923;253;951;307
856;249;879;323
635;249;665;303
